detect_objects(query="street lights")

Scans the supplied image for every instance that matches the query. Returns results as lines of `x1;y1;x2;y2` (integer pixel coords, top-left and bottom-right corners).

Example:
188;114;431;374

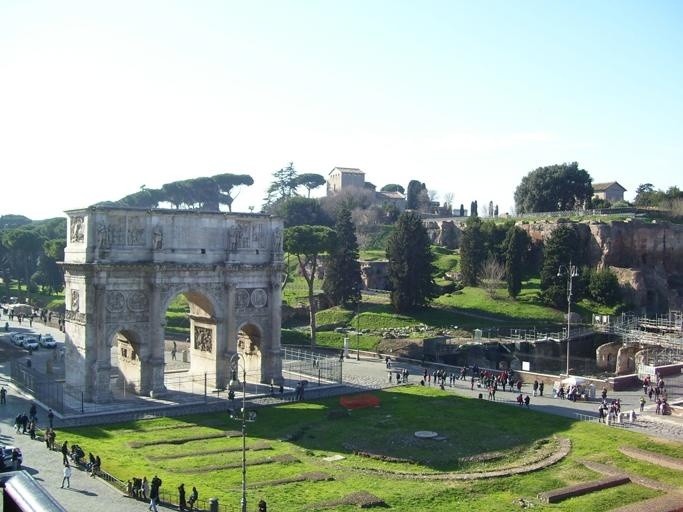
226;350;257;512
556;255;580;375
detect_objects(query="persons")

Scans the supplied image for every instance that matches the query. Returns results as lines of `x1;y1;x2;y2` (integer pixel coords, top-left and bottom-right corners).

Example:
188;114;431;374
385;355;674;423
171;348;177;360
5;304;65;334
270;383;306;401
173;341;176;349
0;387;198;512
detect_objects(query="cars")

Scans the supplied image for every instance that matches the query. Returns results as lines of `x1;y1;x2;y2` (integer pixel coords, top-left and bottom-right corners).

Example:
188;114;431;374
9;330;58;351
0;445;24;468
